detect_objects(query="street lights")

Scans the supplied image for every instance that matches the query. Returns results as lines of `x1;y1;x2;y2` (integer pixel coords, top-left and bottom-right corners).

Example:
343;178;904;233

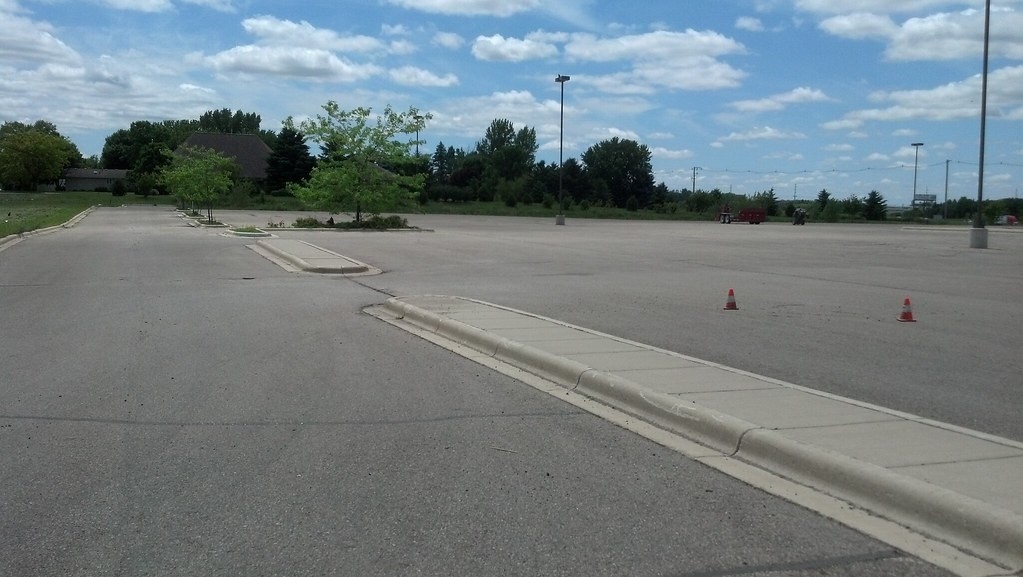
910;142;926;210
554;73;570;225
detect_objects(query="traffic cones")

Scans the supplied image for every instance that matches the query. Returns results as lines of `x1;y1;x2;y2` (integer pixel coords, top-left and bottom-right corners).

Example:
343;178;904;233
724;287;741;312
895;293;918;324
